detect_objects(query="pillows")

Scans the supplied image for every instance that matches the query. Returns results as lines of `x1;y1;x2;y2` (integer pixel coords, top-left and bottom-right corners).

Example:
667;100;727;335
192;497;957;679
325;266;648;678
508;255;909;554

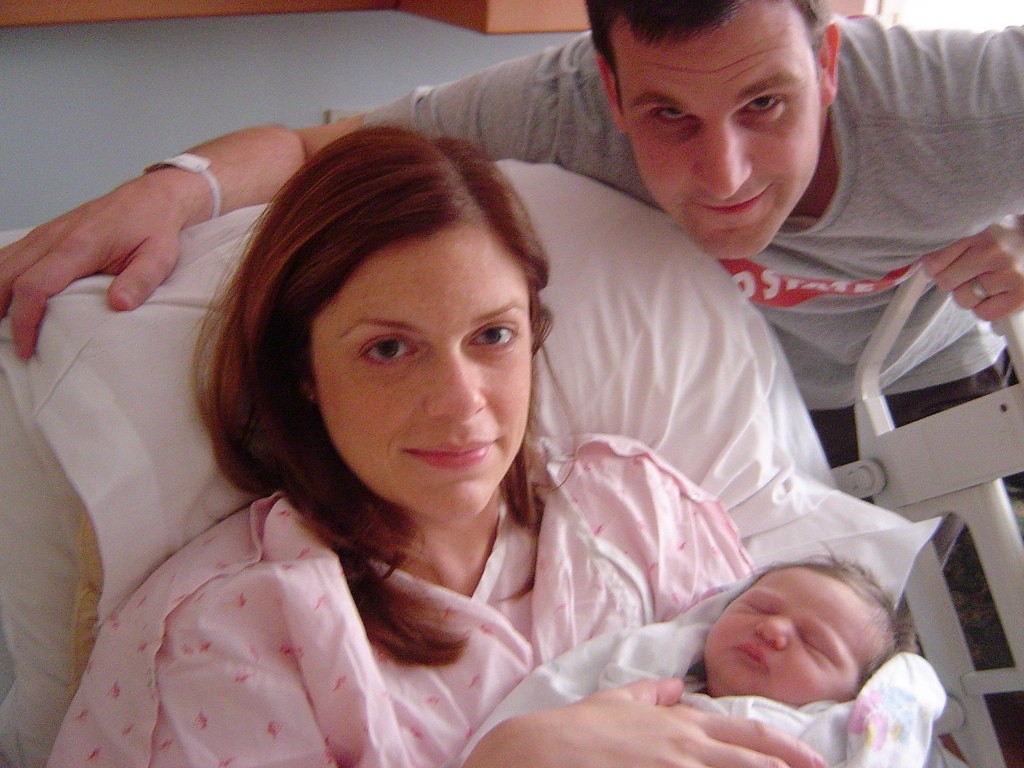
0;159;943;768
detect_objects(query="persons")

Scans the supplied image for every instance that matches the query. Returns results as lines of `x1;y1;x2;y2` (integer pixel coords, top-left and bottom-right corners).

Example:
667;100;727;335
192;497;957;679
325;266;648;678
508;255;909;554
2;1;1024;766
462;555;949;766
44;124;834;764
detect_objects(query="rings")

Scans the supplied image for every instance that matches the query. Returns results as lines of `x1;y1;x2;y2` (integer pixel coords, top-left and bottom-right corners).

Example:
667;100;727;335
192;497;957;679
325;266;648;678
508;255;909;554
969;275;991;301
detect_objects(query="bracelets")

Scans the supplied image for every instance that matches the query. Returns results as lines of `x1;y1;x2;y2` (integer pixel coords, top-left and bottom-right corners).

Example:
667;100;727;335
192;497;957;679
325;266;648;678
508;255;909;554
141;146;223;220
